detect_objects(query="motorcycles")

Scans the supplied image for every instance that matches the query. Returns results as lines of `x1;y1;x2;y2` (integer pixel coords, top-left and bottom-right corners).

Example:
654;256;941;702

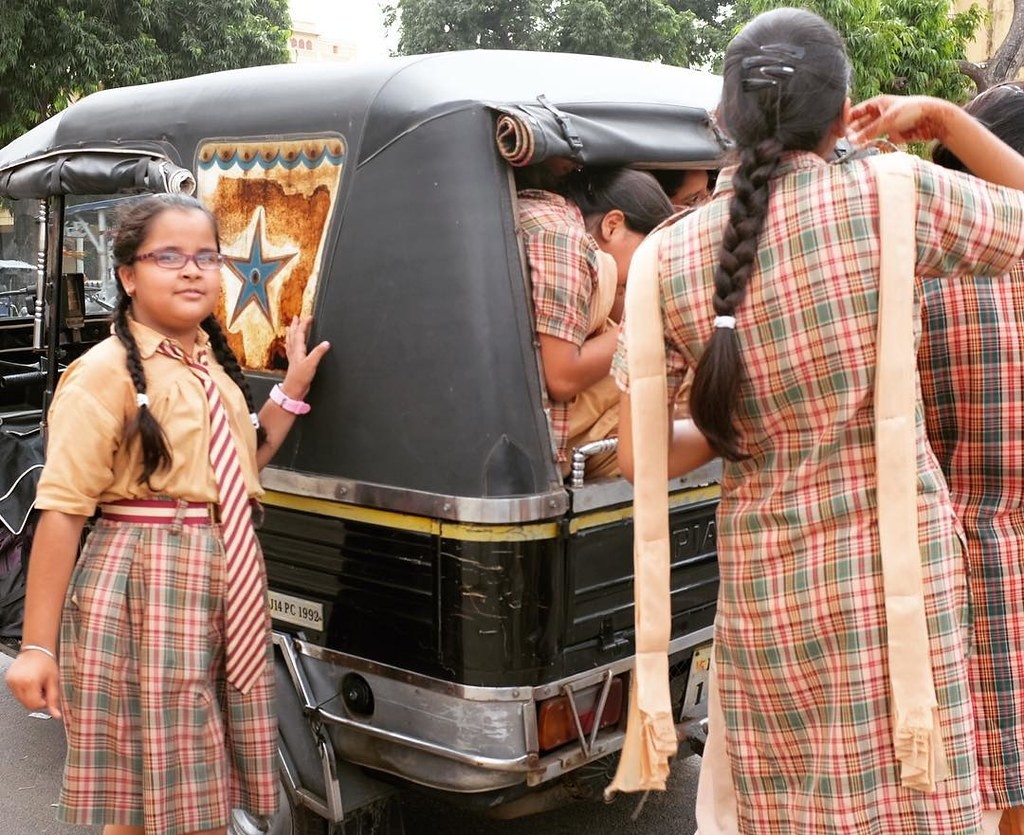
2;47;885;835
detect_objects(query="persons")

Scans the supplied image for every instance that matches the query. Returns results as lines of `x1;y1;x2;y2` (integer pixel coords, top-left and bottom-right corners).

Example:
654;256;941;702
604;7;992;835
515;169;712;486
4;190;330;835
919;81;1024;835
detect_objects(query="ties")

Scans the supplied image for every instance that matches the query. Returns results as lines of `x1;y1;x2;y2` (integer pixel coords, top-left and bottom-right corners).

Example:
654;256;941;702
157;341;269;697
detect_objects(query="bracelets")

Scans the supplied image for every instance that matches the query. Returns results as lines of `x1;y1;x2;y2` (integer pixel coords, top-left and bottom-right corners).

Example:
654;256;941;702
22;645;53;657
270;383;310;415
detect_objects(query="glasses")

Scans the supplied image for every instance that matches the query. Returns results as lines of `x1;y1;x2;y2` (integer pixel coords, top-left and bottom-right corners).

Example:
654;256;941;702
132;251;226;271
676;185;716;207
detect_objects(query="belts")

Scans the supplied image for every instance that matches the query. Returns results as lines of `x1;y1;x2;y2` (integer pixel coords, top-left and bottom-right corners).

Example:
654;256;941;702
97;499;222;526
